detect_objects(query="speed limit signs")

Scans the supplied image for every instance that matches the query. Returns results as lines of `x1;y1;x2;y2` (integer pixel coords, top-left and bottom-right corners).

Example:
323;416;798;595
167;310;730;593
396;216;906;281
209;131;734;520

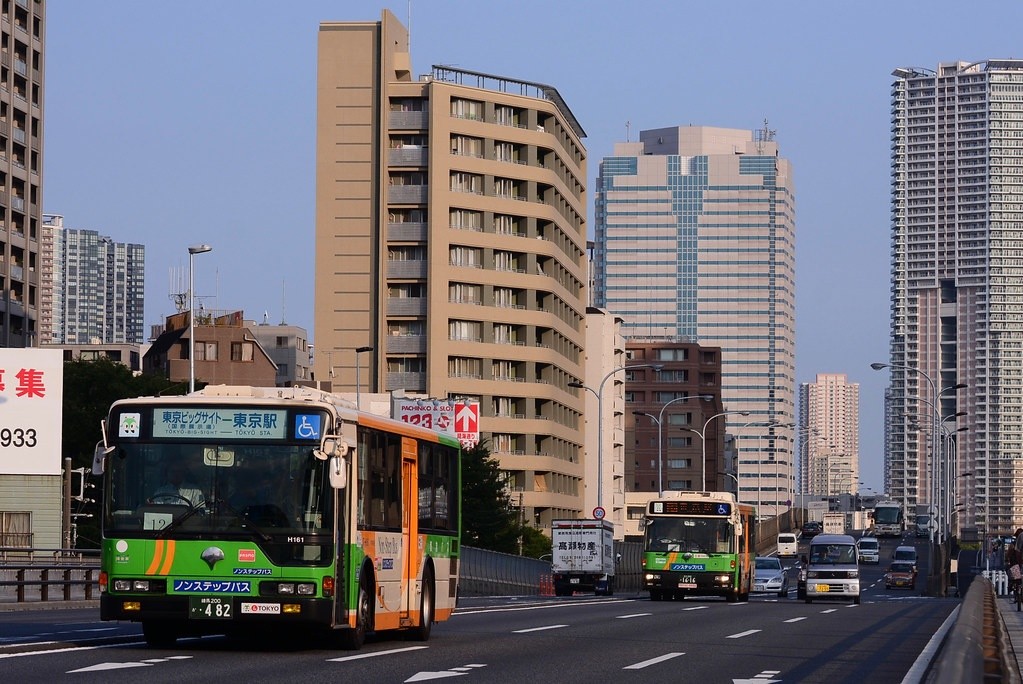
593;506;605;519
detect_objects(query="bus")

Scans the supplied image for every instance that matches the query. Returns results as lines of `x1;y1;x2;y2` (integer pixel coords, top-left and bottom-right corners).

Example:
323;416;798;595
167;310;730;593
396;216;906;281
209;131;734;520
638;490;756;603
805;534;861;604
867;500;905;537
92;383;462;651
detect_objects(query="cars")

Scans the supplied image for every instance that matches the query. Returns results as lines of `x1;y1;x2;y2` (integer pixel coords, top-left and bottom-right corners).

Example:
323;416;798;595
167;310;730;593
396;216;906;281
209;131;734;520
800;521;823;537
753;556;792;598
796;564;805;601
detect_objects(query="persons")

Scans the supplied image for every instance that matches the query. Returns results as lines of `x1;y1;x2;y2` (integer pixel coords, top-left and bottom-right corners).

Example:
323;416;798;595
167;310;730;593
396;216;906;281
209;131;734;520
1005;528;1023;592
144;459;207;509
816;548;832;562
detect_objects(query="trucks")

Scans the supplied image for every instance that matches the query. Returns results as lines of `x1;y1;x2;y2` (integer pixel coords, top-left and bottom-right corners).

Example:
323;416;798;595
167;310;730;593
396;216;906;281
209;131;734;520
551;518;622;596
915;515;930;537
777;532;800;558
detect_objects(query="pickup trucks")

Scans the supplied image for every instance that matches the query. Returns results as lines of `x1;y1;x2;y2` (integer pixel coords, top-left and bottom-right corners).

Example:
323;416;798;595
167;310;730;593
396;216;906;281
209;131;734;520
990;534;1015;542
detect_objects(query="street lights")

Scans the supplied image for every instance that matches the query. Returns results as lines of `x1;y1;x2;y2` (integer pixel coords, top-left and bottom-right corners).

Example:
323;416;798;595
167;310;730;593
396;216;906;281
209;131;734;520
679;408;878;545
355;345;374;411
186;245;213;394
632;394;714;499
568;363;664;519
870;361;972;574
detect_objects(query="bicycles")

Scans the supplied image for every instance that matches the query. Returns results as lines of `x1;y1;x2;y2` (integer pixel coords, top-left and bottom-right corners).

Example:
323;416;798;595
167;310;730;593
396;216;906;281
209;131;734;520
1006;562;1023;612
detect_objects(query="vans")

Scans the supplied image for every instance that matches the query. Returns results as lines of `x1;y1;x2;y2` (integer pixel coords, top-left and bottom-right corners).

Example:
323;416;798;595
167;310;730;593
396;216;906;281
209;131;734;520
859;537;881;564
892;545;918;565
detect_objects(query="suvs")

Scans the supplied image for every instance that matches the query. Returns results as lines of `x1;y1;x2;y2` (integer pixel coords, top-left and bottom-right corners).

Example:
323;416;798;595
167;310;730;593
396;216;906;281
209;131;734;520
884;563;917;590
